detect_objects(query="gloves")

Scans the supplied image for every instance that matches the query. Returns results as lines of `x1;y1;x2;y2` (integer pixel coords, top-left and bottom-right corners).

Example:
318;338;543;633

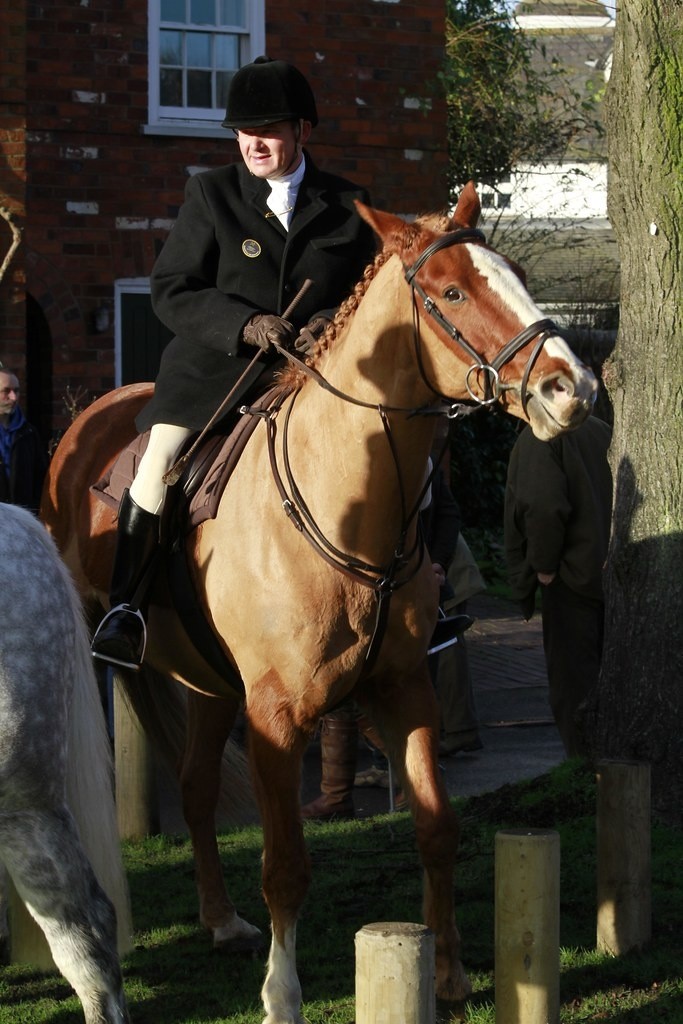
295;317;334;356
240;313;295;355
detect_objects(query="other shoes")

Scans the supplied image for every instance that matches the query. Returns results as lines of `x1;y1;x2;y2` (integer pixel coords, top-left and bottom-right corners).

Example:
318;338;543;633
353;763;388;788
439;728;479;757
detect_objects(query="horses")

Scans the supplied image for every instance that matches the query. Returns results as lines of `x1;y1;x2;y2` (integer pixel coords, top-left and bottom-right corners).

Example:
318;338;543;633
38;179;599;1024
0;504;137;1024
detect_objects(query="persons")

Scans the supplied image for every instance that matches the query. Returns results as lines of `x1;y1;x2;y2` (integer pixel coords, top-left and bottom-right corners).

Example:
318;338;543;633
507;413;614;769
93;56;471;674
0;367;49;518
300;456;475;821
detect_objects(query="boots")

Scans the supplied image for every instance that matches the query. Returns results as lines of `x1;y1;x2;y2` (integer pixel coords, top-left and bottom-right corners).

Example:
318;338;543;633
430;613;474;646
300;719;355;818
93;487;162;663
360;720;445;810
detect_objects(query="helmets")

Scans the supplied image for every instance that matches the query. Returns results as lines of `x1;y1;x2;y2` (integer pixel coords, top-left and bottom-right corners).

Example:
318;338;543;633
222;56;319;128
